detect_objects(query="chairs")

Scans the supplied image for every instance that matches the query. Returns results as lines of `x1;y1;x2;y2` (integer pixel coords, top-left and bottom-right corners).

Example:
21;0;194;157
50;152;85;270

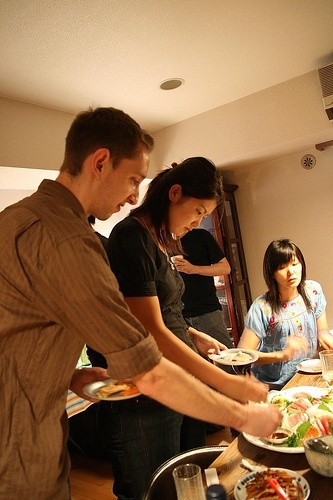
142;445;228;500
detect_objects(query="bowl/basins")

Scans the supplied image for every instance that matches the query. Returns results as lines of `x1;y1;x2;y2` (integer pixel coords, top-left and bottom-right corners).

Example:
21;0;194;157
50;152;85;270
208;348;258;376
304;434;333;476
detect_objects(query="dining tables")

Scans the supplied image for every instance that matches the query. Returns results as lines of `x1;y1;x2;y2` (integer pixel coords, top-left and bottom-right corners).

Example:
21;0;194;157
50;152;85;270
203;345;333;500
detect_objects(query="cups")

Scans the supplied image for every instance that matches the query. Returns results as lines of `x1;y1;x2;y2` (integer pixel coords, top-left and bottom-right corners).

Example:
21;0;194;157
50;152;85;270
171;255;183;271
173;464;206;500
319;350;333;380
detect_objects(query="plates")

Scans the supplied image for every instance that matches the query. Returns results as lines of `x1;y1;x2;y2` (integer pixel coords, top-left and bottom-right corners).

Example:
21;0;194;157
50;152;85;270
263;428;292;446
243;386;333;453
234;468;310;500
84;379;141;400
296;359;322;373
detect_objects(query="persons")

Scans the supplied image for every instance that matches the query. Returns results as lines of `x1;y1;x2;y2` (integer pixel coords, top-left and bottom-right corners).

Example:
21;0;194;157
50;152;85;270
87;156;269;500
171;228;239;437
237;238;333;391
0;108;285;500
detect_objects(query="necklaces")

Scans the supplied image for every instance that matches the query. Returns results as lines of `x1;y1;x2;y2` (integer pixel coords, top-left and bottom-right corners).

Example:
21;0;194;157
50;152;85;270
142;217;175;271
